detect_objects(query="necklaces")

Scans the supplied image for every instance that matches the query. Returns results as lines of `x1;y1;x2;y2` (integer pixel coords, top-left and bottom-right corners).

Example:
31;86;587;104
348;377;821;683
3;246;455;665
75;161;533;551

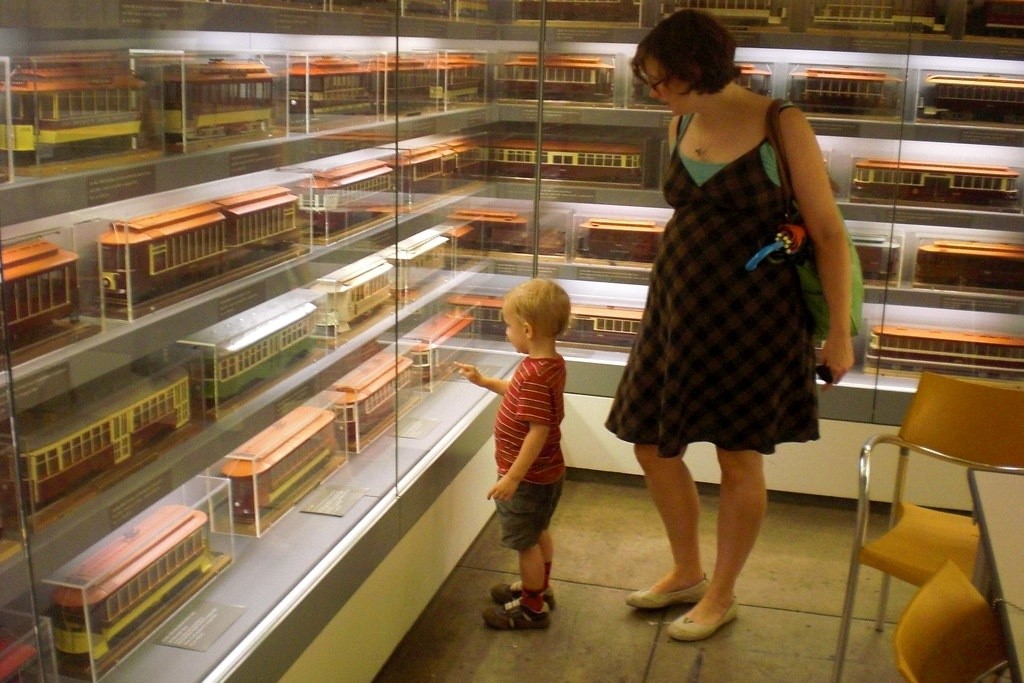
694;148;704;155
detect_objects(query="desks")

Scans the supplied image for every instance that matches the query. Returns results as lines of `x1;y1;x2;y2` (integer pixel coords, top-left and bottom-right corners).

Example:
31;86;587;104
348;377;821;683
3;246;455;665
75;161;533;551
966;468;1024;683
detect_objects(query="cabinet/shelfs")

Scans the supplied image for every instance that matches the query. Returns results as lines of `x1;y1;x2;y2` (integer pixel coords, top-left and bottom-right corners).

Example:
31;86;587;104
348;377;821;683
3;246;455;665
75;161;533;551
0;0;1024;683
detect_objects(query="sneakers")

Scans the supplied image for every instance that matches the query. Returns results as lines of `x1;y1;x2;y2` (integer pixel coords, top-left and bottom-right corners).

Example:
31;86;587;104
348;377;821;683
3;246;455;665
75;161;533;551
490;580;555;611
483;597;552;629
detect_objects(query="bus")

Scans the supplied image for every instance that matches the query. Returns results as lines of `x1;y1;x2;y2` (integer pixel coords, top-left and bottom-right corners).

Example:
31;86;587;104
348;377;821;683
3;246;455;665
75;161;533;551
0;0;1024;683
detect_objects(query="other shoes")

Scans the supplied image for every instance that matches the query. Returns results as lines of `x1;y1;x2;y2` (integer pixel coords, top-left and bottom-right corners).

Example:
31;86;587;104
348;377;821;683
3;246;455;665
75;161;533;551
626;573;711;608
667;595;738;640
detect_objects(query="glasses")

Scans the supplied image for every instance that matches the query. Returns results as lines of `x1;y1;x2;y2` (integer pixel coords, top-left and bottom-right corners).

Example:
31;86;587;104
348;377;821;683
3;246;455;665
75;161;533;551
645;73;673;96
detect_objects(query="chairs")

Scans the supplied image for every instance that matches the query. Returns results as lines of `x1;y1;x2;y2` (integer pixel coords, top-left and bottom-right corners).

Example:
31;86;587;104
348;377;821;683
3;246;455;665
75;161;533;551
831;371;1024;683
893;559;1008;683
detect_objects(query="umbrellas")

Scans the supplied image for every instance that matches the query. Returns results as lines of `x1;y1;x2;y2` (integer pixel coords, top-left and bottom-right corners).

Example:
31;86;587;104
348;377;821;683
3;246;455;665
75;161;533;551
745;222;806;271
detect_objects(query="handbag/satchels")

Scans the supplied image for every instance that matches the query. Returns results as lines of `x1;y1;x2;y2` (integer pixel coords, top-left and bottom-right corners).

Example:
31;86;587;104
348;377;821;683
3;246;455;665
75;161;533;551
765;98;863;340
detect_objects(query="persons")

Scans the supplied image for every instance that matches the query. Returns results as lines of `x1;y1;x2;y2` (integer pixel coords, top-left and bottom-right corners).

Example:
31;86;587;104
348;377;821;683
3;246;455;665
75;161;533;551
604;9;855;640
454;278;571;632
